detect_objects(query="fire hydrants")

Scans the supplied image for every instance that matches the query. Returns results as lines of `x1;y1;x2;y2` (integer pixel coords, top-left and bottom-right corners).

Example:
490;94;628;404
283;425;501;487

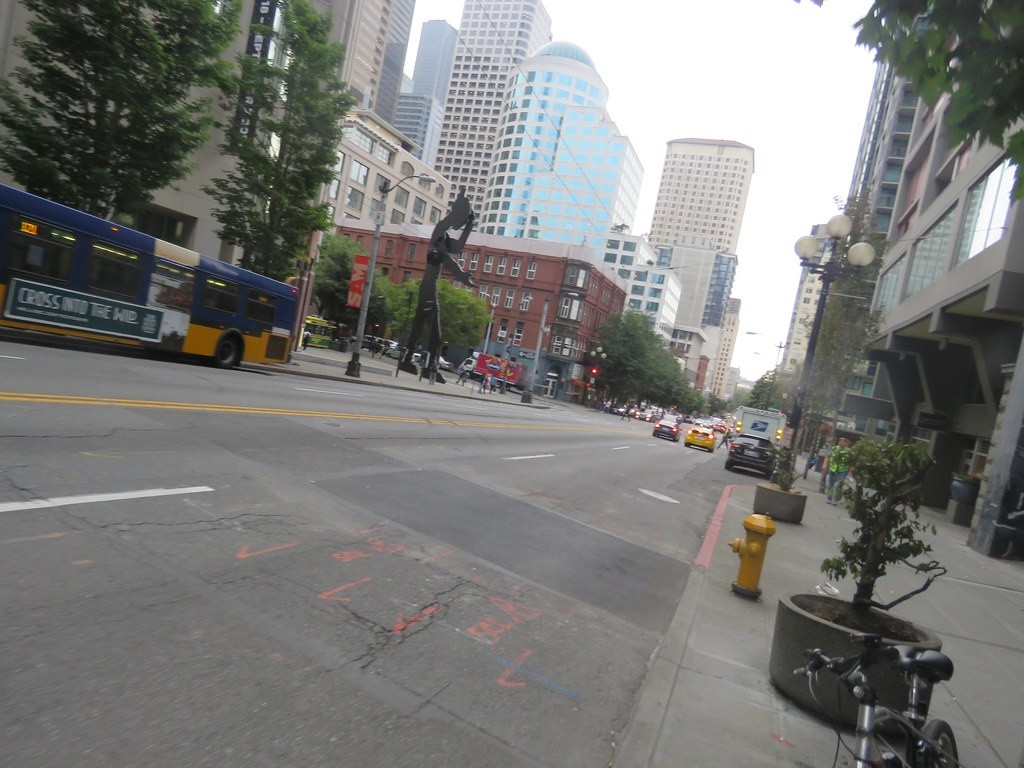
727;513;776;597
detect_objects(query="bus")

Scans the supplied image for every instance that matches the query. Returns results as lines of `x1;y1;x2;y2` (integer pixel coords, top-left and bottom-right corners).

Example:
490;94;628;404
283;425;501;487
301;314;337;348
0;182;300;369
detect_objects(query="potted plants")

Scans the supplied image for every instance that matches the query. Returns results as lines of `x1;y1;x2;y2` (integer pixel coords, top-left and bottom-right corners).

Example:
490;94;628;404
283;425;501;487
952;471;980;503
769;438;948;735
753;446;808;523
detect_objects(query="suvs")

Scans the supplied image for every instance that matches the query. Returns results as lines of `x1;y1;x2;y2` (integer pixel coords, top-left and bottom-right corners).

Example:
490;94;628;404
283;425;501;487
724;434;776;481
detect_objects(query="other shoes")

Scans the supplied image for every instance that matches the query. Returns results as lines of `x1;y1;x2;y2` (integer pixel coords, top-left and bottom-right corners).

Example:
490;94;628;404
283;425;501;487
825;499;832;504
832;501;837;506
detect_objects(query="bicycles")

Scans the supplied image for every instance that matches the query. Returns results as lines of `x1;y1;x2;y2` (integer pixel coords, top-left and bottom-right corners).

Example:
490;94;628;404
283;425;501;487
794;633;960;768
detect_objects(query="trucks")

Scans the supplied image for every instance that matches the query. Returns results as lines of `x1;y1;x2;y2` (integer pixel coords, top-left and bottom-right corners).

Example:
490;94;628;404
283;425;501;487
735;406;786;444
457;351;528;391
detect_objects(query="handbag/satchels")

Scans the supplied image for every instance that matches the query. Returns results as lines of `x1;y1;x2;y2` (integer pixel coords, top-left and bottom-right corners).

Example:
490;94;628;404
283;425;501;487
841;473;858;494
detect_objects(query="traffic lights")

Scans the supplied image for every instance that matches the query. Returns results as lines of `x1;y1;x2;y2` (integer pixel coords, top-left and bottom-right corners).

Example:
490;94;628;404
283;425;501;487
591;369;597;374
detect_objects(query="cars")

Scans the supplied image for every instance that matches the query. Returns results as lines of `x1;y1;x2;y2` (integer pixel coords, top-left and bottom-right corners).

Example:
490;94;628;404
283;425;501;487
611;402;733;432
684;426;718;452
350;335;453;370
653;420;682;442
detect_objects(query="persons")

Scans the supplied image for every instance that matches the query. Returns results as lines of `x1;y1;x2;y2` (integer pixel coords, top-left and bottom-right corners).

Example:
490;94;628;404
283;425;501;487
621;402;630;422
456;366;465;386
371;340;385;358
823;437;854;505
601;399;611;413
478;373;497;394
304;329;311;350
717;429;730;449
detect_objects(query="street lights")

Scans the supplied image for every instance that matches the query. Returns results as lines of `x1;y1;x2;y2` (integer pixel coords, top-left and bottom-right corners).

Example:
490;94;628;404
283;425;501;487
746;331;785;409
754;214;876;523
583;347;607;407
344;174;437;377
